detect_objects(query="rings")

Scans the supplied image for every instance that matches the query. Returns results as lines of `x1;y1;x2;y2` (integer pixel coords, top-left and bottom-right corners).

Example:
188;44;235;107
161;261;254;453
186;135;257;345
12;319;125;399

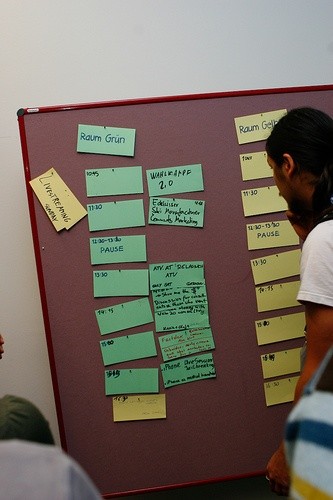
265;475;271;481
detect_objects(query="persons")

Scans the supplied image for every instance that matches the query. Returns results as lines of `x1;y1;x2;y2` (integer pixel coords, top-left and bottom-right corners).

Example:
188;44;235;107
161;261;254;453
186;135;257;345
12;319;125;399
0;395;104;500
265;107;333;497
284;391;333;499
0;335;4;360
